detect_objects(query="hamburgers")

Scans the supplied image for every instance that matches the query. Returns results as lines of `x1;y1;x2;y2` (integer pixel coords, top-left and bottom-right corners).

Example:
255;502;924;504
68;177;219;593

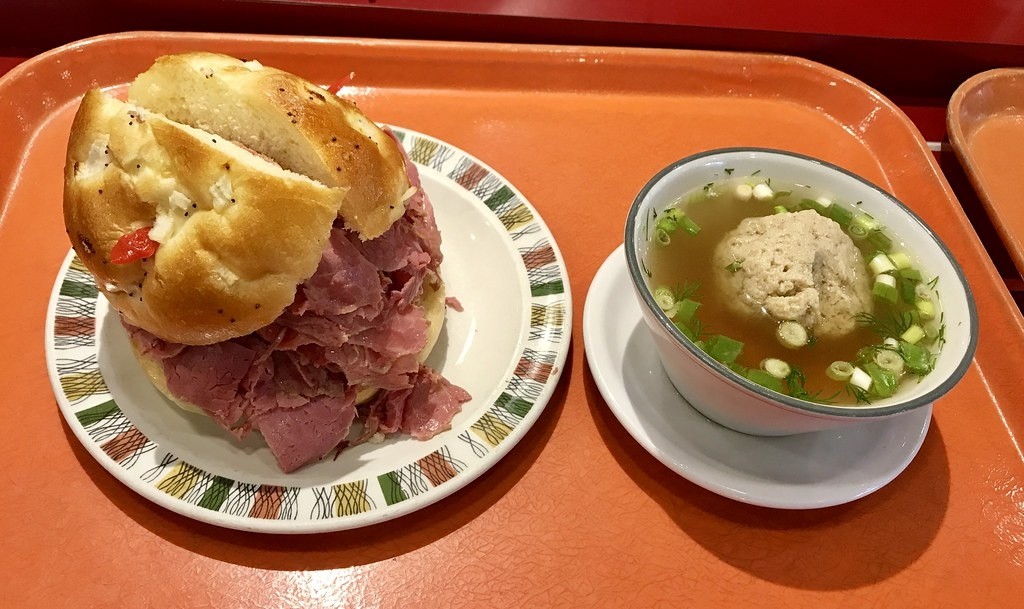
58;48;474;472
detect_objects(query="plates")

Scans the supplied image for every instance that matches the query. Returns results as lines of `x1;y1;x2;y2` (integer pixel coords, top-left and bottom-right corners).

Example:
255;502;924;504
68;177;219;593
44;121;573;536
583;241;935;510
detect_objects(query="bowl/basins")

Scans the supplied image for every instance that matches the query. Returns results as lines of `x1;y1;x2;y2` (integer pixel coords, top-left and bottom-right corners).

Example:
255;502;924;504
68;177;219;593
624;146;980;437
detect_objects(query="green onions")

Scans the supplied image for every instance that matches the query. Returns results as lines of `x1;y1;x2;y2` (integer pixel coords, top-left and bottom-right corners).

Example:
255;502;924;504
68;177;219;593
650;180;940;406
